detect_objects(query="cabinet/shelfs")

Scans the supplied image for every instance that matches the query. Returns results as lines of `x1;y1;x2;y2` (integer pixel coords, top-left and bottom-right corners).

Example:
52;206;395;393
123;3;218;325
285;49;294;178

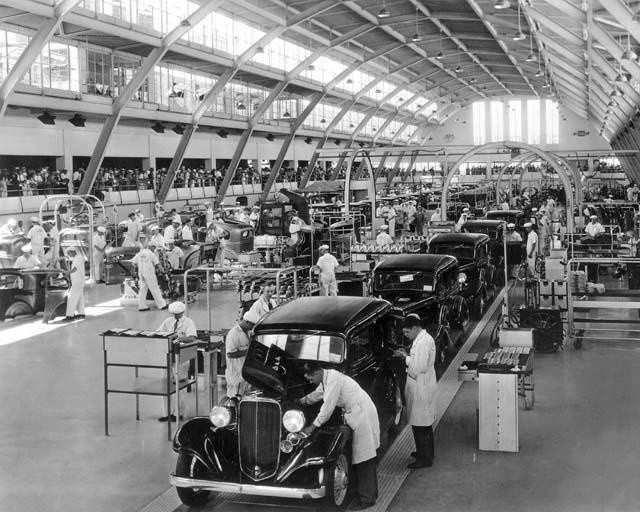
100;331;195;442
566;254;640;352
179;264;308;416
38;193;106;288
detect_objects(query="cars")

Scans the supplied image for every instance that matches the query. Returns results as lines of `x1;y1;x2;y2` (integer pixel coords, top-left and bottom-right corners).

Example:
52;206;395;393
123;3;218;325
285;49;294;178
0;267;72;321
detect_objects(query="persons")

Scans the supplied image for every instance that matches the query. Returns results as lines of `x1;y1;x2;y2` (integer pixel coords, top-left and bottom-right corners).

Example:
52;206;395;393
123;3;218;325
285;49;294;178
0;161;640;321
295;363;381;511
392;313;437;469
225;311;261;400
156;301;198;422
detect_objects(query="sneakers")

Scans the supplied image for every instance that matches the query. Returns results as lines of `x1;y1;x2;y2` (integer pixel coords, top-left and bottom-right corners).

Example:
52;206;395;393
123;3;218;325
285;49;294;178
159;414;183;422
161;304;169;311
62;314;86;322
407;461;433;469
410;451;436;459
350;493;378;500
349;501;375;511
138;307;151;312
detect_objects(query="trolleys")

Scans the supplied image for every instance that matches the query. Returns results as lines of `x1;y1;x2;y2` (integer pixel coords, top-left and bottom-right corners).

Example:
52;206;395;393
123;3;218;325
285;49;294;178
563;206;640;351
518;275;564;354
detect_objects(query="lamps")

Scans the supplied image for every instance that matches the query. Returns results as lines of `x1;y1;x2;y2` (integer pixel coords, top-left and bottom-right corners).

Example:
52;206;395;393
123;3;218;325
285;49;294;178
220;0;639;145
69;114;86;129
36;112;56;125
152;123;165;134
172;125;185;136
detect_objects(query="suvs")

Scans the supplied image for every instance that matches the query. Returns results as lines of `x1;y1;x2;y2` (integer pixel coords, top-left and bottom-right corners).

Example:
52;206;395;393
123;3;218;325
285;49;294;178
460;220;527;286
361;253;465;350
169;296;413;511
482;210;537;256
428;232;497;319
